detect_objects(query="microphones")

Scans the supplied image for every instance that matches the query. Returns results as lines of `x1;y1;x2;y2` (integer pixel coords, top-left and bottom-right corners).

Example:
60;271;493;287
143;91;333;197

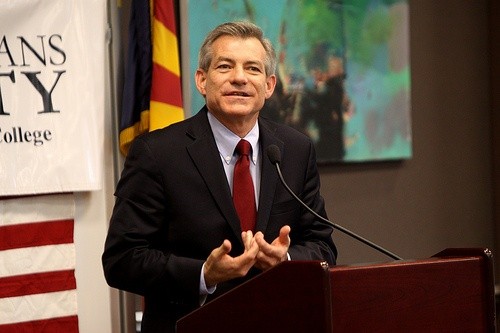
266;144;404;262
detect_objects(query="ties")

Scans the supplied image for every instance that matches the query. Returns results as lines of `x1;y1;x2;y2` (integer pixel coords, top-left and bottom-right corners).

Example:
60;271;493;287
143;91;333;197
232;139;258;237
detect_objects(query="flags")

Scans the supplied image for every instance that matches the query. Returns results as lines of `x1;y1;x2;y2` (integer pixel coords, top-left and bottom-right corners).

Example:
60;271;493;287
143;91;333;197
119;0;184;158
1;193;79;332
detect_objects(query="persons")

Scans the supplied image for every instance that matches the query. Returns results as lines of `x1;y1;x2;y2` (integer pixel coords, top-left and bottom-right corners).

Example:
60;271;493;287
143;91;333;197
102;20;339;333
